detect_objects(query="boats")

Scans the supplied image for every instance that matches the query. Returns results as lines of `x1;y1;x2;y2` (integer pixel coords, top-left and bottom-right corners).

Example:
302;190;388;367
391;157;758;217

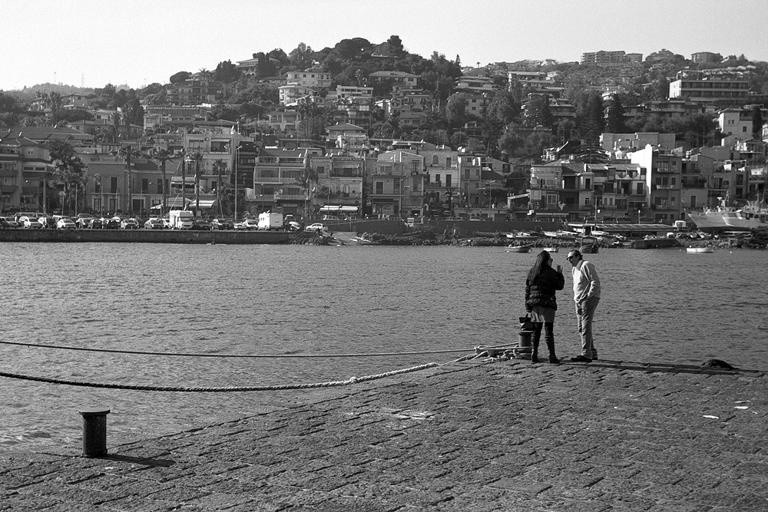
571;234;600;254
542;243;561;254
502;239;534;252
719;188;768;235
504;226;628;242
684;195;743;236
684;243;715;253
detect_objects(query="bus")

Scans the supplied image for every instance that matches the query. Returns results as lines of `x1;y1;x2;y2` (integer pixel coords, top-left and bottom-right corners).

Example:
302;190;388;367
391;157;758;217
534;212;569;225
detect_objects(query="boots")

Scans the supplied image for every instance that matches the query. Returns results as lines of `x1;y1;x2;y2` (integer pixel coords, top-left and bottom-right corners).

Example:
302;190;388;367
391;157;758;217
549;349;560;364
530;348;542;363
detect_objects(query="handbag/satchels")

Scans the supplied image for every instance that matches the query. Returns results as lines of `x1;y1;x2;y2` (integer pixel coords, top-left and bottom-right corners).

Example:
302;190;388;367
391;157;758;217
519;315;535;334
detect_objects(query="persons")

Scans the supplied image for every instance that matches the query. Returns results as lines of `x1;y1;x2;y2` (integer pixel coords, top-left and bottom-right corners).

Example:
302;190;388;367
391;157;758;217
524;250;565;364
567;249;601;362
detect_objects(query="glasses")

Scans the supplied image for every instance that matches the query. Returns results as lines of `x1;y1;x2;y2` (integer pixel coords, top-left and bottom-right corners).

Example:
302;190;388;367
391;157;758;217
565;255;574;261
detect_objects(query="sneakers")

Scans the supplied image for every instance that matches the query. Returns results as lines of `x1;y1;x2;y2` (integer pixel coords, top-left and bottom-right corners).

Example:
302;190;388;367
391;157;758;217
570;353;593;363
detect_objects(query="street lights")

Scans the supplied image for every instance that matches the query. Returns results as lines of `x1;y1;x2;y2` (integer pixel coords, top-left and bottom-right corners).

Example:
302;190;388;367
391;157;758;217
323;151;336;221
226;122;241;223
298;148;308;220
68;153;81;218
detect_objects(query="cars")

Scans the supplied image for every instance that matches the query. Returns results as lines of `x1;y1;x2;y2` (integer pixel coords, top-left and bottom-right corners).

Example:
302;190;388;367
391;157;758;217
468;215;487;223
642;231;719;241
